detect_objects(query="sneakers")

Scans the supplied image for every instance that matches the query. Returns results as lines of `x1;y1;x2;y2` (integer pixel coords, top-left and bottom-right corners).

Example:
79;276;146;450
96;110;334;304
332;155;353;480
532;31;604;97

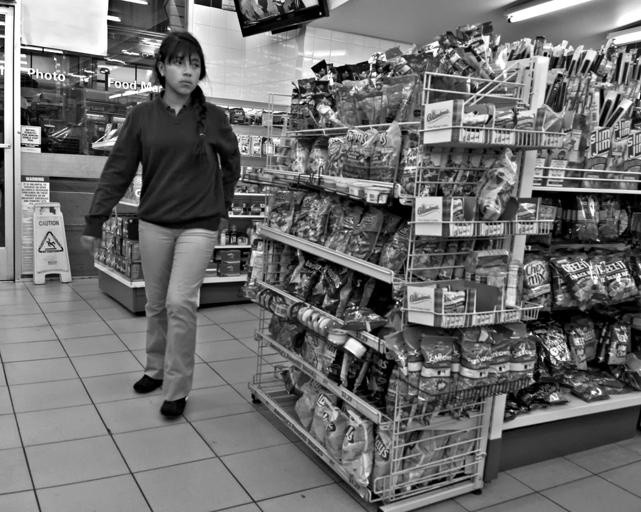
160;396;188;417
133;375;163;393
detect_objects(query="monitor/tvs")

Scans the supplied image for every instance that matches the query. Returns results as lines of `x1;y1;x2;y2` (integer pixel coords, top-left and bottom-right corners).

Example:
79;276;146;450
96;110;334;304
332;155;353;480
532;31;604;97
233;0;329;37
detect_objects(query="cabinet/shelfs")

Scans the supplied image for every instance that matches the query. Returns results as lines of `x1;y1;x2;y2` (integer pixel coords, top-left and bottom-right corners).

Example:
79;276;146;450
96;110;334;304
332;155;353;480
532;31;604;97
89;56;641;511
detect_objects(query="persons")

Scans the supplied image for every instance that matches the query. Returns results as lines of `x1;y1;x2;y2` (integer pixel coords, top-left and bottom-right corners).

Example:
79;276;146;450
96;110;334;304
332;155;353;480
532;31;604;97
80;31;241;416
